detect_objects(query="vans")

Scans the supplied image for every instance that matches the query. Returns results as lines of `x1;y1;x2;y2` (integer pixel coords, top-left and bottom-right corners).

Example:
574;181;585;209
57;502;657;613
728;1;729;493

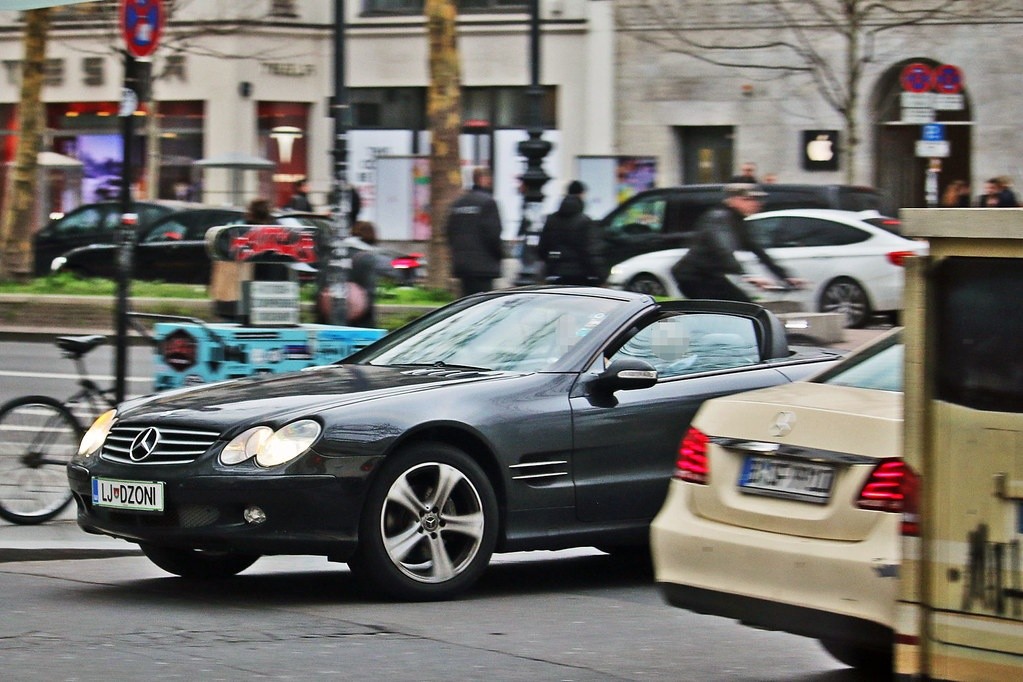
514;183;897;285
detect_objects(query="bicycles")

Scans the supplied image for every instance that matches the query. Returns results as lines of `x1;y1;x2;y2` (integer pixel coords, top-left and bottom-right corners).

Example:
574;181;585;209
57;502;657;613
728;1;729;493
0;333;117;525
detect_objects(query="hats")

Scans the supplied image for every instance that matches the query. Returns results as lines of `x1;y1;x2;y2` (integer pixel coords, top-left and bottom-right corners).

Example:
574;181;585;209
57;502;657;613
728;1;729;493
722;183;770;197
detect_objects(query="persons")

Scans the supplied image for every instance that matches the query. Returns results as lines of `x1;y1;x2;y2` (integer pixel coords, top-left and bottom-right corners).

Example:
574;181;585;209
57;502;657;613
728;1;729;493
731;161;777;184
285;178;313;212
306;214;399;327
537;180;607;284
329;174;362;225
445;167;502;297
242;198;293;280
941;175;1018;207
672;187;805;303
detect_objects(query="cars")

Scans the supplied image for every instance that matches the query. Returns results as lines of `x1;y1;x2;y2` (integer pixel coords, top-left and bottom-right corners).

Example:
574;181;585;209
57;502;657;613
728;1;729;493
647;328;906;668
607;208;929;326
28;200;197;276
47;208;429;284
64;287;856;602
